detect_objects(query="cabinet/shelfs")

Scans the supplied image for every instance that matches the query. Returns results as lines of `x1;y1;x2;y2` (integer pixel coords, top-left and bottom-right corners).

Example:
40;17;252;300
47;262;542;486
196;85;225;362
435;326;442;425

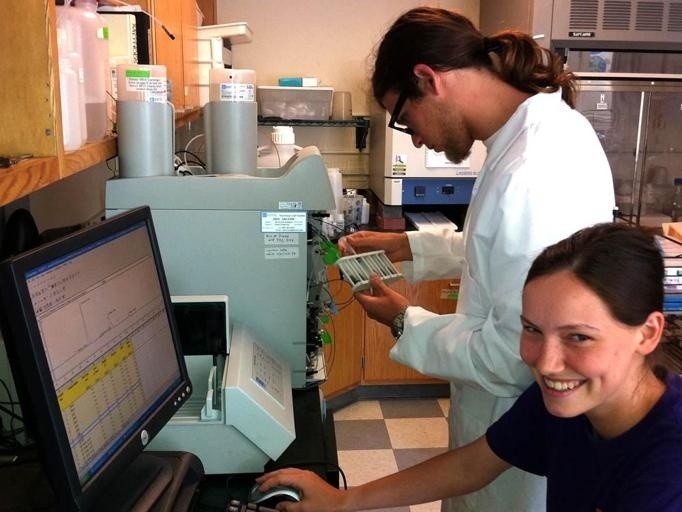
314;246;463;416
0;2;219;210
565;72;682;235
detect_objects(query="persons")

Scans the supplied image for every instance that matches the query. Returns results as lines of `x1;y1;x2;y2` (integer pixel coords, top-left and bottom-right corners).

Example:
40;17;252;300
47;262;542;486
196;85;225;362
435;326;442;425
335;7;616;511
252;221;682;512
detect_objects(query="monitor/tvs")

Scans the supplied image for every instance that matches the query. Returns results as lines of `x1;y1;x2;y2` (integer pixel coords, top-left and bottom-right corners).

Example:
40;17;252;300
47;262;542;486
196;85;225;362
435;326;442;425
0;205;195;512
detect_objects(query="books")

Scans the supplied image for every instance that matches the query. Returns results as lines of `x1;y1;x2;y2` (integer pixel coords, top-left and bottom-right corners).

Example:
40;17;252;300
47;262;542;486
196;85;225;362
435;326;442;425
654;221;681;314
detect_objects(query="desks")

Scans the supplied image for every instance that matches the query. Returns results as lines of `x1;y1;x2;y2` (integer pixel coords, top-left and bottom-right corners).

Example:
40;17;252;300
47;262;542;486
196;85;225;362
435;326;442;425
191;381;338;511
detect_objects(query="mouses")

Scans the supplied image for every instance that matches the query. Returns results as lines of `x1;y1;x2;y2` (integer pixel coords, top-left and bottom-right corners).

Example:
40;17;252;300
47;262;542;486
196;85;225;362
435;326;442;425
245;483;300;505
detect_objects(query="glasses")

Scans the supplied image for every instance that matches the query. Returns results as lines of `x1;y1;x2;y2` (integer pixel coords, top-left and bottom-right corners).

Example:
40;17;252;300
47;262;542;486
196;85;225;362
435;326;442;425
387;74;418;136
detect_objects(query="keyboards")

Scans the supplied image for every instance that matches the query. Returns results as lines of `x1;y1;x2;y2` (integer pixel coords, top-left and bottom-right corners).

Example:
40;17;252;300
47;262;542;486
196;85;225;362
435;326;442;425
226;498;282;512
